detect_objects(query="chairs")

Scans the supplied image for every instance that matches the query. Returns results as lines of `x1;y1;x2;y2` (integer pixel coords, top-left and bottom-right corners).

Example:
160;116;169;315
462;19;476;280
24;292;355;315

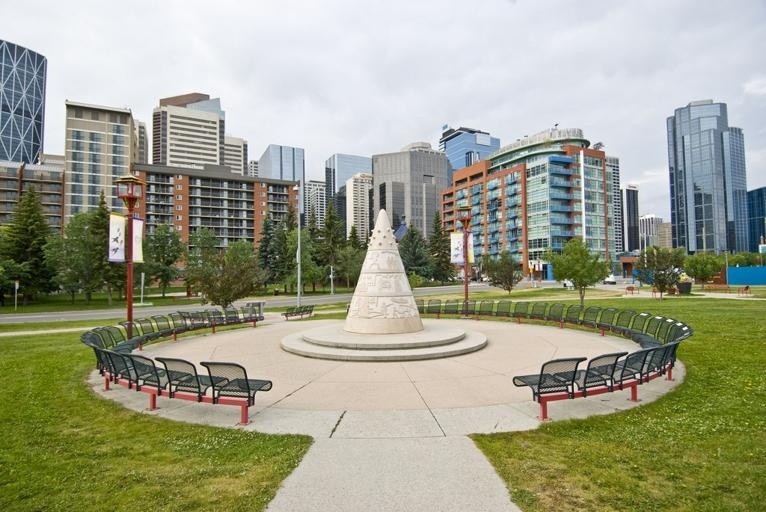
512;340;695;420
548;300;692;340
125;353;273;424
417;300;547;333
80;310;190;390
191;303;263;329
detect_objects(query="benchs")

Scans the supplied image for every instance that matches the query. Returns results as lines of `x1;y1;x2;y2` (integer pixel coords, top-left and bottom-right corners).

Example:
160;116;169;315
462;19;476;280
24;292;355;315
281;305;315;320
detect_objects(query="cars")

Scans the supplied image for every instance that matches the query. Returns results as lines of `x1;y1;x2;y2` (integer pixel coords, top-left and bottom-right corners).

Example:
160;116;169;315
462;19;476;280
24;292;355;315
604;275;616;284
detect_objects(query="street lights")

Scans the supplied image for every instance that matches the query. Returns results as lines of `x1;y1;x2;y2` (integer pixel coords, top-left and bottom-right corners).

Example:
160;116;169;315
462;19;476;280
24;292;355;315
457;205;473;317
725;251;728;284
112;175;148;340
293;185;301;307
